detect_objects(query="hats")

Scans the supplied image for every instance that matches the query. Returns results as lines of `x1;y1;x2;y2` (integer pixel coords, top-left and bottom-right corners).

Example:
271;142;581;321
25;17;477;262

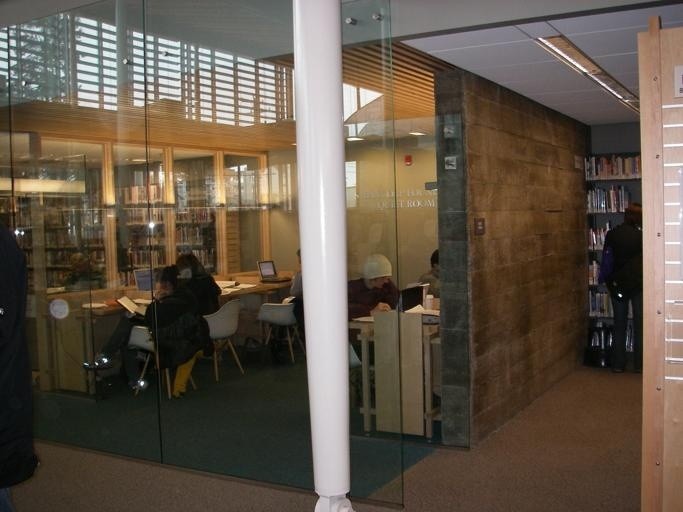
360;252;394;281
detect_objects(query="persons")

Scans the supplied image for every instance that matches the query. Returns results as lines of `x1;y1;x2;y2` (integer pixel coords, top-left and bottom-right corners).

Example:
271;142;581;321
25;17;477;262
80;263;193;392
0;215;41;489
348;253;401;365
174;252;221;316
279;248;301;314
417;248;439;298
596;201;643;373
200;209;216;264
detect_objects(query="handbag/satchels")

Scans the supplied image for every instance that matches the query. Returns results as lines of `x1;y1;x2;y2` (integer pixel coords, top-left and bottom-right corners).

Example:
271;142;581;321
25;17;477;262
605;254;641;303
582;327;611;370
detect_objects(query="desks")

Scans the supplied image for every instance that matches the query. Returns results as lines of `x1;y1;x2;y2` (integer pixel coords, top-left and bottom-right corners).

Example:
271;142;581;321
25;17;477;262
48;269;297;400
348;314;440;444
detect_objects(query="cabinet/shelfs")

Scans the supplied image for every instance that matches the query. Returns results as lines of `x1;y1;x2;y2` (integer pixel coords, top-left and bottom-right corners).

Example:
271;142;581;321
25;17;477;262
584;175;642;373
0;162;241;295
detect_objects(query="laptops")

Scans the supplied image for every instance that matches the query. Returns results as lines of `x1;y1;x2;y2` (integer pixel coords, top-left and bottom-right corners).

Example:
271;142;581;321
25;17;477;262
257;260;292;283
133;269;156;293
399;286;423;313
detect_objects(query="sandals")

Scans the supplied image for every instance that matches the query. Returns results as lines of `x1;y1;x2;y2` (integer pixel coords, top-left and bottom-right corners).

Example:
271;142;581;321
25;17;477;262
81;357;113;371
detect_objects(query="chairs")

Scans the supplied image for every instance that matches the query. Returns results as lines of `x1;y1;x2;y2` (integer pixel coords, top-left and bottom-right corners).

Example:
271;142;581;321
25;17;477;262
127;295;307;400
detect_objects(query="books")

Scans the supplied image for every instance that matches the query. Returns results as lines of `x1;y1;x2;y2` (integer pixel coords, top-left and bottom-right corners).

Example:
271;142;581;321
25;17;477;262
582;153;643;353
0;180;212;314
214;279;240;289
